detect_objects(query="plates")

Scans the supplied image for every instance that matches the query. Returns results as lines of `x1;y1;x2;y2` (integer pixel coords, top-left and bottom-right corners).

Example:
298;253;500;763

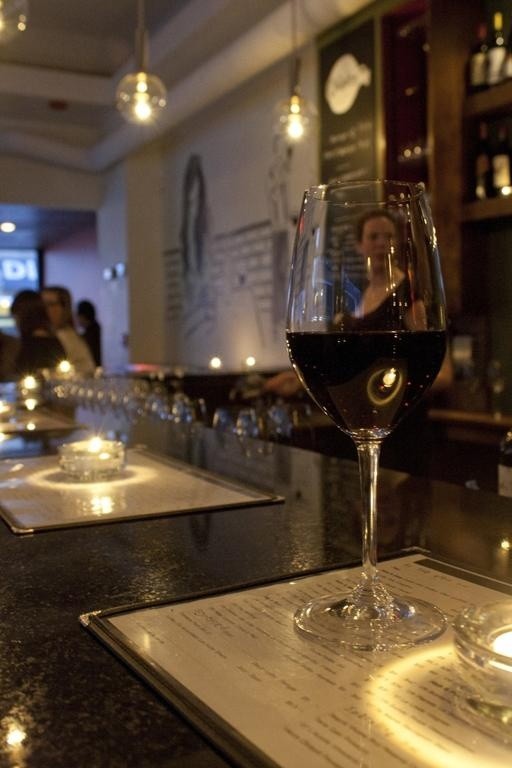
448;600;512;711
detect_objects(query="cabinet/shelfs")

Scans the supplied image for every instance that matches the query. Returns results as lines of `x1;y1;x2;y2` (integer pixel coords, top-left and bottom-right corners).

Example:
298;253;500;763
429;10;511;230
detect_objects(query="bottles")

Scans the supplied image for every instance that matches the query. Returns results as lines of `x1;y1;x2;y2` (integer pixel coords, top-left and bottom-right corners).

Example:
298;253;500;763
497;430;511;501
463;11;512;199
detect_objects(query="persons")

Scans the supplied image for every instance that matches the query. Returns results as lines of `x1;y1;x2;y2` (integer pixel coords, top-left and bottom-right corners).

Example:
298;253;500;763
0;289;67;384
177;152;219;369
263;209;453;394
1;286;96;380
75;298;101;370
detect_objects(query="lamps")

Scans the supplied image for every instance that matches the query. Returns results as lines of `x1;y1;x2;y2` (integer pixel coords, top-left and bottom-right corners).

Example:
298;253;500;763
105;1;174;127
268;3;322;147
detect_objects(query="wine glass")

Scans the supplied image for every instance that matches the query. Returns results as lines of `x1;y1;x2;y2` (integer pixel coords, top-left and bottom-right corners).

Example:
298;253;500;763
34;362;285;494
484;356;506;421
283;177;452;654
